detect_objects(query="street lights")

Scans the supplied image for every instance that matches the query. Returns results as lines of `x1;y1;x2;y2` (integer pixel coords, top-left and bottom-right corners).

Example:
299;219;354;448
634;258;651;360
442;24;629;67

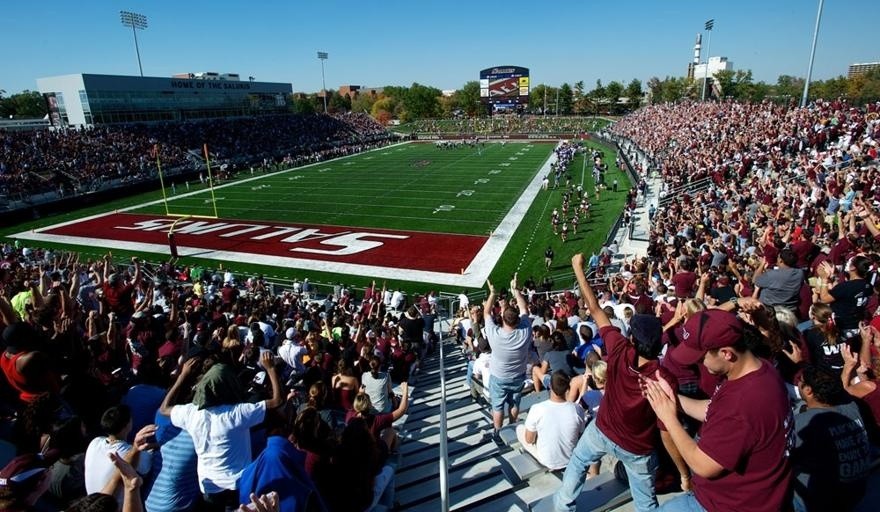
701;18;716;102
119;9;148;79
317;52;331;115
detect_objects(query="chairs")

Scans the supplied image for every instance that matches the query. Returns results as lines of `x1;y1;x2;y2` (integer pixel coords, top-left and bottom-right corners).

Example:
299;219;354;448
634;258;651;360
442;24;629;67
459;311;858;512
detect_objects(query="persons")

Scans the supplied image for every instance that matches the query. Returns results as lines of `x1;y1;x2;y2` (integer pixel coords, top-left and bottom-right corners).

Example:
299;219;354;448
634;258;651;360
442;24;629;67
0;95;880;512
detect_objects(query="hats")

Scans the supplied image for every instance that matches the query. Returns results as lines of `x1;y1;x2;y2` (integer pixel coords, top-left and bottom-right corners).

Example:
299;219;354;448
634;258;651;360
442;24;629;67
629;313;662;343
285;327;297;339
668;309;744;366
195;322;208;336
1;324;27;353
